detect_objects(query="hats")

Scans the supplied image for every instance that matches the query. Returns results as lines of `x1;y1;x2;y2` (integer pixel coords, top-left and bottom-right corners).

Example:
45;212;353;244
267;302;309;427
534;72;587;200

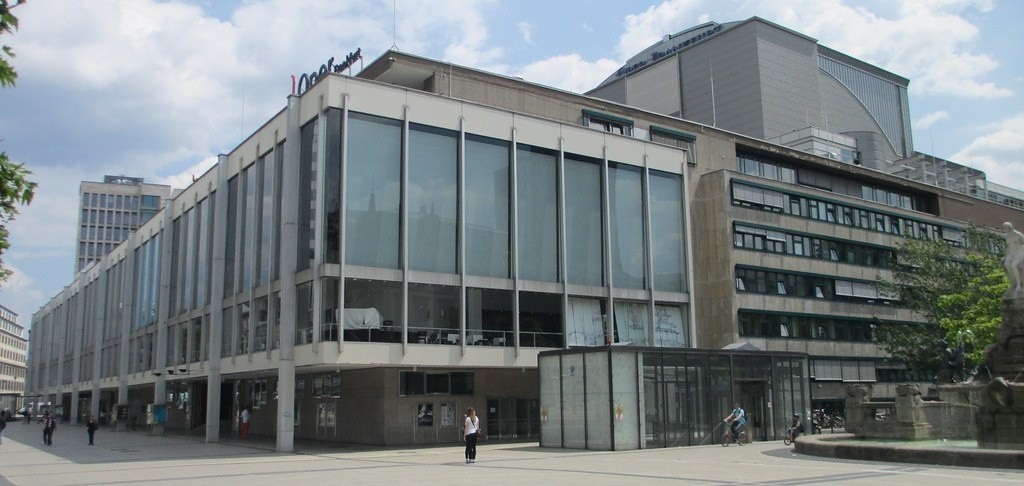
794;414;799;418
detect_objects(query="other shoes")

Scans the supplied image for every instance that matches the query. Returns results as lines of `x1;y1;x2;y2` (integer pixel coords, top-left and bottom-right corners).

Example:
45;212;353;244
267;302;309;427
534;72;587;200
466;458;468;462
467;460;475;462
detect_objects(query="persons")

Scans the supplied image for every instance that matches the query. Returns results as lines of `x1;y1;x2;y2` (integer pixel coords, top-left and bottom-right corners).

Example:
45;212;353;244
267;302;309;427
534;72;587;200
789;413;800;442
86;415;98;445
22;409;31;424
99;408;106;424
240;406;249;439
40;410;55;446
0;411;6;444
463;408;479;463
723;403;745;443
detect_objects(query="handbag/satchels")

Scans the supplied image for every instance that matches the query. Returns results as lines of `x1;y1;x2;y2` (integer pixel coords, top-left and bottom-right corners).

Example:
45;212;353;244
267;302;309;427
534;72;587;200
94;421;98;429
812;419;819;424
476;428;482;437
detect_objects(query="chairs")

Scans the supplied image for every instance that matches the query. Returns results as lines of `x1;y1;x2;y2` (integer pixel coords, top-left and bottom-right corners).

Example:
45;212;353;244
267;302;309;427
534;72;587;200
416;331;493;345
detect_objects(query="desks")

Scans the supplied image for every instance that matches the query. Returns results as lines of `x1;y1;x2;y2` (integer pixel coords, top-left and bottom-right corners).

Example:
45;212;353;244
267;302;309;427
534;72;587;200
493;337;507;346
447;334;460;344
467;335;483;345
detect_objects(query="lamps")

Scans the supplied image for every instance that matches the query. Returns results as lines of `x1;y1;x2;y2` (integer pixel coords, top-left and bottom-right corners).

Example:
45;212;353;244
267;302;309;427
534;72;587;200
153;373;169;376
167;370;188;376
413;365;418;372
521;367;526;373
179;369;204;373
336;366;342;373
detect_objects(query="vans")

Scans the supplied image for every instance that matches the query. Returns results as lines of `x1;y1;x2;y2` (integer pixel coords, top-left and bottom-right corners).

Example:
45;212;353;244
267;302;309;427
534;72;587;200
16;401;52;416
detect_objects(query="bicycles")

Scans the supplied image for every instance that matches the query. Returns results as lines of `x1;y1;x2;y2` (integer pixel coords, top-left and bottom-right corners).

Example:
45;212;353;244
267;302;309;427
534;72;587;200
783;408;847;446
718;418;750;450
